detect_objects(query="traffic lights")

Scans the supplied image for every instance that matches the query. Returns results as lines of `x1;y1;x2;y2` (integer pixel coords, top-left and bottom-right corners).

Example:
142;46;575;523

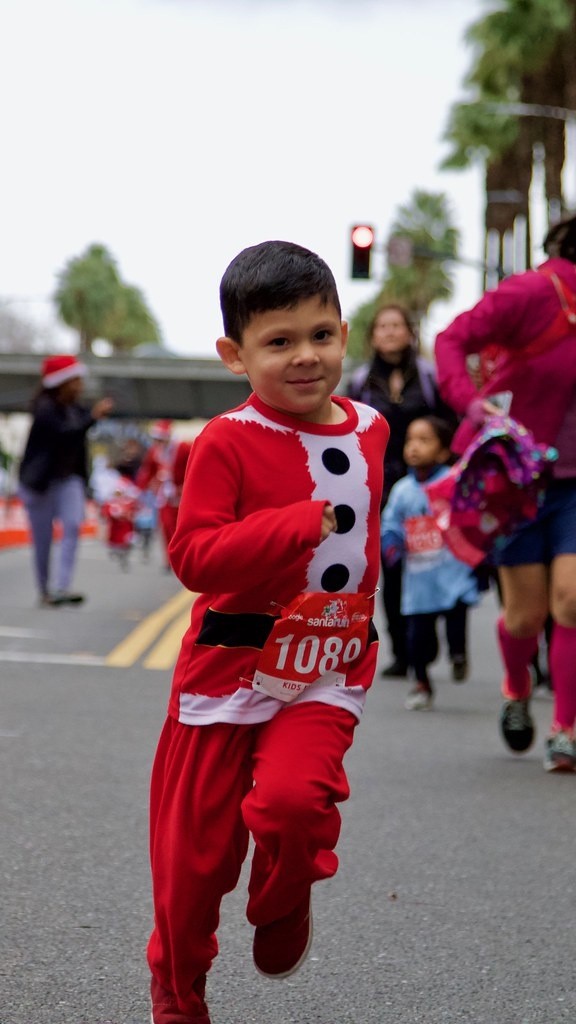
350;226;374;280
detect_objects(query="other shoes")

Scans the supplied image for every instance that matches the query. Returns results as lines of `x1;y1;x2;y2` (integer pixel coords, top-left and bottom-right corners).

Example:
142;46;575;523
49;590;83;606
37;592;60;609
451;658;468;681
405;690;432;711
382;662;409;677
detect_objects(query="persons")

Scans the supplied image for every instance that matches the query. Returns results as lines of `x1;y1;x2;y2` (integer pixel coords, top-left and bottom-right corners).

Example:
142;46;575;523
89;414;192;576
13;353;114;607
345;302;558;710
148;241;391;1024
429;213;576;776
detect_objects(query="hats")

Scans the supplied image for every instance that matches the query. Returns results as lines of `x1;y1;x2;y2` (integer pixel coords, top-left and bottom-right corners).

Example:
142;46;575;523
148;418;173;439
41;355;86;388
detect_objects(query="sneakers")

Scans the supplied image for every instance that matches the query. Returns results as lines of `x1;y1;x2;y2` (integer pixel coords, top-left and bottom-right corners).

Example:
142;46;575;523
543;730;576;771
500;697;536;755
252;885;314;979
